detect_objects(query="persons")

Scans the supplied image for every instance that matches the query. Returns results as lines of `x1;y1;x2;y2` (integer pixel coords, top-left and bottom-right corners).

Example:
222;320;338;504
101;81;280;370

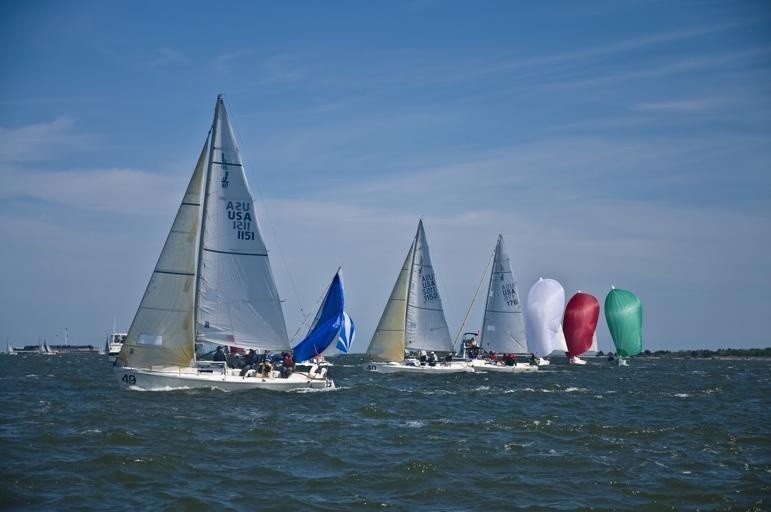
531;354;539;365
314;353;321;365
466;337;519;366
194;342;295;379
409;349;454;368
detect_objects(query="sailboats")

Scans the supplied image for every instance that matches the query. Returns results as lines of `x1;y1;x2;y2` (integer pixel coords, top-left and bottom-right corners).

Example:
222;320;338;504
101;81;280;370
527;275;567;366
468;230;540;372
604;285;645;366
564;289;601;364
361;217;475;373
113;93;347;391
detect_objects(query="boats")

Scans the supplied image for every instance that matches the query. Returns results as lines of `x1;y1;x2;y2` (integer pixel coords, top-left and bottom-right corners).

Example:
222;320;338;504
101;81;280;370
294;355;334;367
6;336;102;355
456;332;480;362
106;332;127;363
